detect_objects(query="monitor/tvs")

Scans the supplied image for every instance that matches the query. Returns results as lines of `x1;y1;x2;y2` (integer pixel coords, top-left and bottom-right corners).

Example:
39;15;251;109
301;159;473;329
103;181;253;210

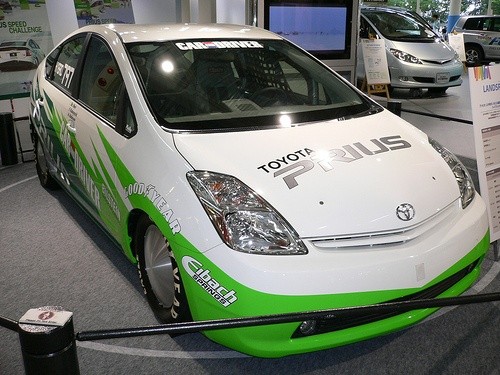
264;0;353;60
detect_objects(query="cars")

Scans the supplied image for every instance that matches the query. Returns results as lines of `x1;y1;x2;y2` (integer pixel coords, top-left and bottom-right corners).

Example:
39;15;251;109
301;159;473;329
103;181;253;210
0;38;40;69
358;6;462;86
451;14;500;67
28;22;492;357
